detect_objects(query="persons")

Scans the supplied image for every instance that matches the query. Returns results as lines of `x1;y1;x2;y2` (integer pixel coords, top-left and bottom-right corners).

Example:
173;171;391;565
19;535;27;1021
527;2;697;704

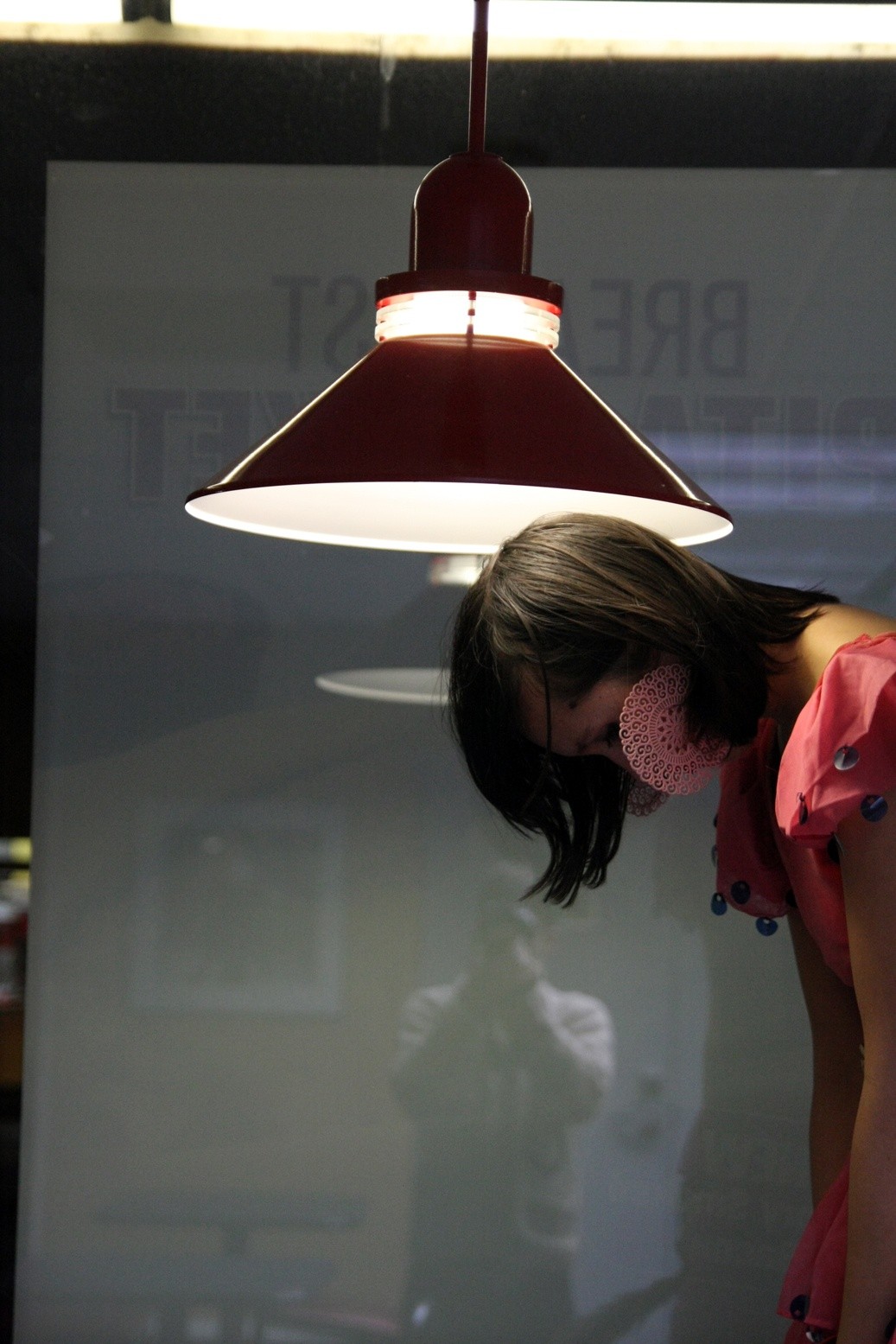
391;863;612;1344
449;515;896;1344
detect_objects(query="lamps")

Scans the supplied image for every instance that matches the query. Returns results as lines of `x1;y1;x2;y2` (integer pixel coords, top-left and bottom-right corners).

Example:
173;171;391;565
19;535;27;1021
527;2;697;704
316;551;488;704
183;0;736;550
